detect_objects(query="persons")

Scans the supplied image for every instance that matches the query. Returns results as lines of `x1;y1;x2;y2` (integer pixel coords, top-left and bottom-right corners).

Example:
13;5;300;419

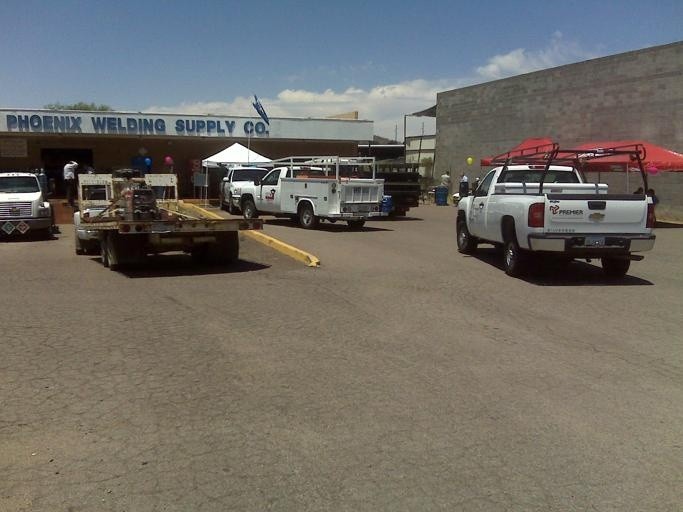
62;158;79;208
632;187;659;229
459;173;480;187
440;170;450;199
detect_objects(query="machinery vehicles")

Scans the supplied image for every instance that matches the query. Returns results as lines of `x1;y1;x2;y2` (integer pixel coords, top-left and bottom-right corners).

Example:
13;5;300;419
70;167;266;276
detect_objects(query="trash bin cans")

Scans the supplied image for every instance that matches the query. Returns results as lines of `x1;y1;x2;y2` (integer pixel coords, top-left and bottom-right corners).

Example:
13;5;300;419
435;185;448;206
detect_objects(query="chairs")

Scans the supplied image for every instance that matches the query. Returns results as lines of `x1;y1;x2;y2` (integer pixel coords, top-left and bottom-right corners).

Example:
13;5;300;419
506;175;529;182
552;173;578;183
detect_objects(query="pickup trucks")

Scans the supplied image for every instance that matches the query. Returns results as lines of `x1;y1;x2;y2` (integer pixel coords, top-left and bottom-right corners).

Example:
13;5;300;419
454;140;658;279
240;151;393;231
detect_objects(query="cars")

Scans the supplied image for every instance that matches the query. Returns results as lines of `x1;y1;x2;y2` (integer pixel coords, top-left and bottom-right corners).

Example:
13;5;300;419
0;171;54;238
217;165;271;213
